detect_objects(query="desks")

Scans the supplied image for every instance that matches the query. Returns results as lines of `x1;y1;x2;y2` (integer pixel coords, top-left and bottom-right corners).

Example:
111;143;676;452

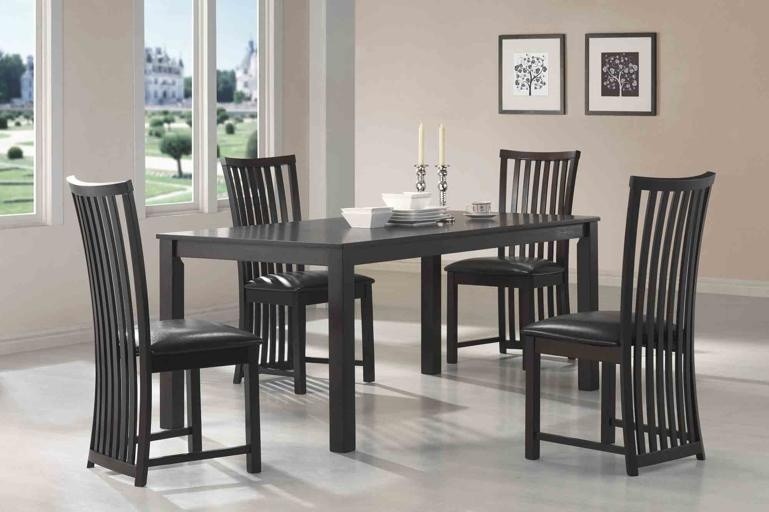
155;204;602;454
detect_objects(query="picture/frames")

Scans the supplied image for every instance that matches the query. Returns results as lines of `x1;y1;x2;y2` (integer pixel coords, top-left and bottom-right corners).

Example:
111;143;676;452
583;31;659;119
496;32;566;116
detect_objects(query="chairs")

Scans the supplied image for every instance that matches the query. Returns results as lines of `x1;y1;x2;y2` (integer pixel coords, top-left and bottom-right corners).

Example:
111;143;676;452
442;147;585;371
65;174;268;490
219;155;377;395
519;171;718;476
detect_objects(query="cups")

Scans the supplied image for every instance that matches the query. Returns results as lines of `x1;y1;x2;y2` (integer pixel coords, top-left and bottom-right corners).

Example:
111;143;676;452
473;202;490;215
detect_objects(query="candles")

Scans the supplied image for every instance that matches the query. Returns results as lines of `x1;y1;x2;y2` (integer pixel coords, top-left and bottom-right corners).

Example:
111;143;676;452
438;124;447;166
418;119;426;165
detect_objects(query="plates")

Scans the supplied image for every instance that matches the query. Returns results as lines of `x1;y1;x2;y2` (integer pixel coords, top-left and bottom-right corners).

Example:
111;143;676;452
340;192;448;230
463;214;496;220
